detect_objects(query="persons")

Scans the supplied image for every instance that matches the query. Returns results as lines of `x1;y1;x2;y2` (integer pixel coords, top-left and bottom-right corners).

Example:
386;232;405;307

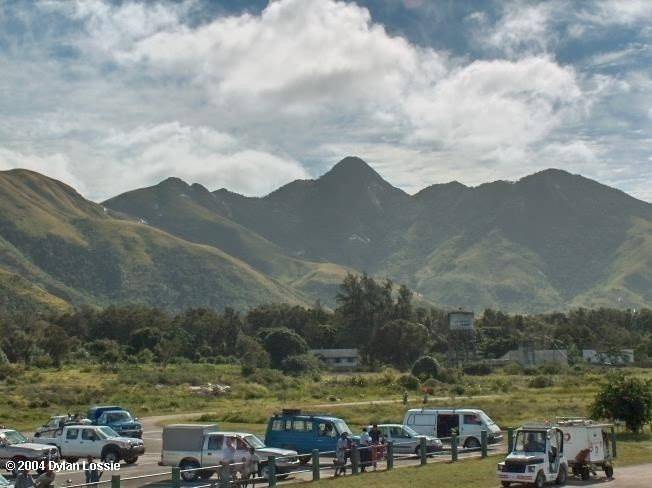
333;422;383;477
35;463;56;487
218;437;260;488
541;432;553;462
14;467;37;488
403;389;407;405
422;391;428;405
570;447;590;481
84;454;103;488
0;421;7;428
66;411;83;423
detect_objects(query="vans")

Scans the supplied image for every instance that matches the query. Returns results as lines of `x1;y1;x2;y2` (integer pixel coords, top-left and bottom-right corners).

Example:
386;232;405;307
402;409;503;449
264;413;362;459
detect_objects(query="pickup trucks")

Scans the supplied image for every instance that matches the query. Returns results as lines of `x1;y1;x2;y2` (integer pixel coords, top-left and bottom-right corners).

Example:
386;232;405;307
158;431;301;483
1;405;145;478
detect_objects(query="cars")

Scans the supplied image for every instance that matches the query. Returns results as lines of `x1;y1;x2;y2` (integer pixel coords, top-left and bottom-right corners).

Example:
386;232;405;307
366;424;442;456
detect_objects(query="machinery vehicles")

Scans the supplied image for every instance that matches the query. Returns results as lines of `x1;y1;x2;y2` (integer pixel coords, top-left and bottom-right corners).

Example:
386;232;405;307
497;416;617;487
